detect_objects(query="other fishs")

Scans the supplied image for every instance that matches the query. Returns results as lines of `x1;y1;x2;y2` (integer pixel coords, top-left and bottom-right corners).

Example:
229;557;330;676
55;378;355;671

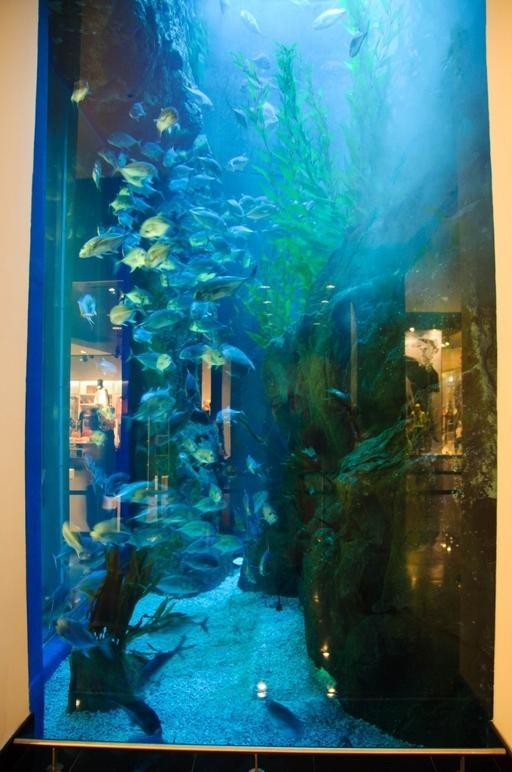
51;78;282;744
